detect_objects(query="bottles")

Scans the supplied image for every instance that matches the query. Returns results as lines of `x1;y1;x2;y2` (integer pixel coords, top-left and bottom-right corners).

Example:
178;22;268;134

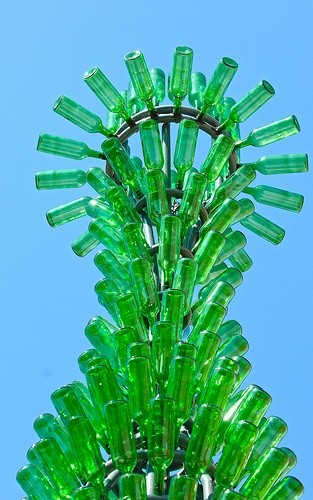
15;44;308;499
